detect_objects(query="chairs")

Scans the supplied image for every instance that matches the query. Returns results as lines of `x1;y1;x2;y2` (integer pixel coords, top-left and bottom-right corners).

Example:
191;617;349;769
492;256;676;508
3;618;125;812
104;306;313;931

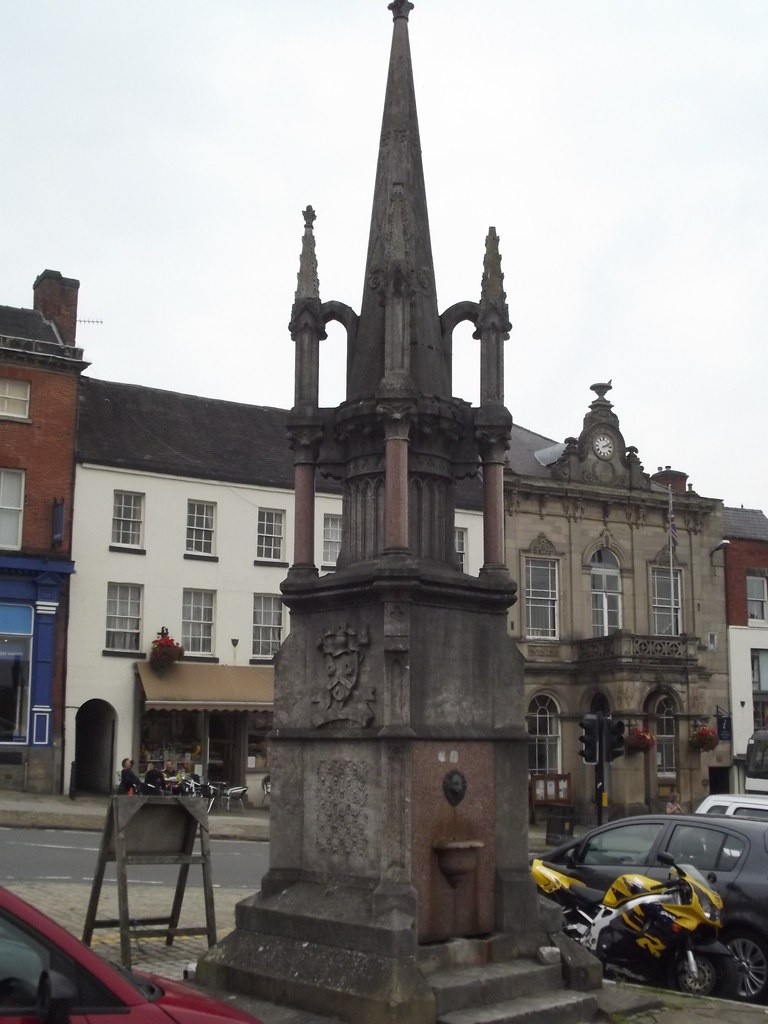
675;834;712;869
131;774;271;814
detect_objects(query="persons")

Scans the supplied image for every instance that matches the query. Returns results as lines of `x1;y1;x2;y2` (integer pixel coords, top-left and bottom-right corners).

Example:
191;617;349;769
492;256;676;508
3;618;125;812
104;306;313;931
164;759;178;778
145;763;168;795
117;758;142;795
666;793;684;814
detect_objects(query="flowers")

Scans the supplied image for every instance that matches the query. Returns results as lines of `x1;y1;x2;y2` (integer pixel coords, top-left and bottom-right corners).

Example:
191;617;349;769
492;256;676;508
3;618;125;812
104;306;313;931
148;626;183;671
625;727;656;755
687;724;721;752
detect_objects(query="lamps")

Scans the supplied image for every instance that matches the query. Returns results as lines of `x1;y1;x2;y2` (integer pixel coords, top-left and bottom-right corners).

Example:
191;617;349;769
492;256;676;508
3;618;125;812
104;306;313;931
710;540;731;557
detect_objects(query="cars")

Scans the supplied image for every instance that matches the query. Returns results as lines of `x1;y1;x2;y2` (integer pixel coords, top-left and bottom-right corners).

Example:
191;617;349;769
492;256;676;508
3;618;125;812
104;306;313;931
528;814;768;1004
694;792;768;859
0;884;261;1024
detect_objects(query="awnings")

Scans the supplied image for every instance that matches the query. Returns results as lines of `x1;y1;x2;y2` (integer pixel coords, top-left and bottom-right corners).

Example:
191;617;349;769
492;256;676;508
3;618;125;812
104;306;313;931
136;660;276;714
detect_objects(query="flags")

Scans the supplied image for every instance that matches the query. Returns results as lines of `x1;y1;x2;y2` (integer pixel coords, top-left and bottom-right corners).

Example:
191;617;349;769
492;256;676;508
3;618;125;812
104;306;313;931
668;491;679;552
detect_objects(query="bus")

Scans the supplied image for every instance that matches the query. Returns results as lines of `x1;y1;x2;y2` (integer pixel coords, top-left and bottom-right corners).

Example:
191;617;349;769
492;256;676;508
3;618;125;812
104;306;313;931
744;729;768;796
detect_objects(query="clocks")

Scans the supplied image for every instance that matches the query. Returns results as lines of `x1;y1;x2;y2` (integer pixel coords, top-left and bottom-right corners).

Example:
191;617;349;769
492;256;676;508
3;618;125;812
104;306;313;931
592;431;615;461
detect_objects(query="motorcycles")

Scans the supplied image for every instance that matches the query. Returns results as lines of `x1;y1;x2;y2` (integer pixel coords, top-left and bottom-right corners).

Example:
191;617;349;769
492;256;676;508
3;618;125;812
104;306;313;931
531;851;734;997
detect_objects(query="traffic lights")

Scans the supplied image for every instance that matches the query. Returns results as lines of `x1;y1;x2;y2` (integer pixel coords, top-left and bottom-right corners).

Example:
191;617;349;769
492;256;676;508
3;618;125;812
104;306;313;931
579;714;599;766
601;720;625;762
